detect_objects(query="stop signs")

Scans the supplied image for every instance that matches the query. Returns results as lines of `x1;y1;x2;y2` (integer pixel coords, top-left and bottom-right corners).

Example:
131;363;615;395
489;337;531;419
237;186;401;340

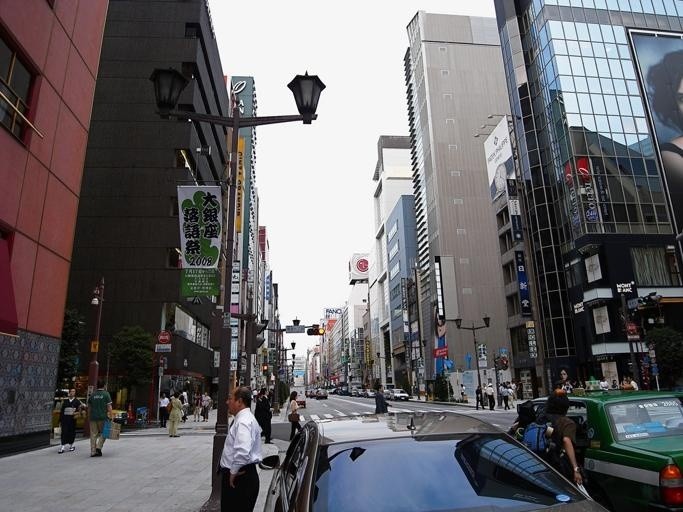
626;322;635;334
158;354;163;366
157;330;170;344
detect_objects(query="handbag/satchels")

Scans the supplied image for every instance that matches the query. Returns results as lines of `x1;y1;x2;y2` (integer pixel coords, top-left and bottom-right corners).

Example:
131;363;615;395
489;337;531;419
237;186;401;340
103;421;121;440
166;402;172;413
288;411;299;422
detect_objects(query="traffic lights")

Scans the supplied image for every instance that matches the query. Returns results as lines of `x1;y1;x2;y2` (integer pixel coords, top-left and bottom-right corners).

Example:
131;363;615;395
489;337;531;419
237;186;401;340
263;364;268;375
245;321;267;355
637;294;660;307
499;358;508;370
306;329;325;335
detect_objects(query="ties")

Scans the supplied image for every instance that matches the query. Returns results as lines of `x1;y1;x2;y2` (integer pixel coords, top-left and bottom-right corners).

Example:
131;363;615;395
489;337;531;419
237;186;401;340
215;419;235;476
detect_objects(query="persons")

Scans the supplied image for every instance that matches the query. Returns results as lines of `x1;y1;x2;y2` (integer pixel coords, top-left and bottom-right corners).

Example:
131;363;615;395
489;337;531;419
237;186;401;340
57;387;81;453
158;386;212;439
537;388;582;487
215;386;261;512
288;391;301;440
644;48;682;226
474;381;523;411
515;407;547;451
459;384;465;397
553;367;578;394
87;380;113;457
374;385;391;414
599;375;640;391
250;388;273;445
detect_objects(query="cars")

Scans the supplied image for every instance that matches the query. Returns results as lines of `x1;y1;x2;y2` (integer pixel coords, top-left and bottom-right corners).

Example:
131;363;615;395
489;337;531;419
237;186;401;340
507;389;682;511
257;412;609;511
295;383;409;409
51;397;129;433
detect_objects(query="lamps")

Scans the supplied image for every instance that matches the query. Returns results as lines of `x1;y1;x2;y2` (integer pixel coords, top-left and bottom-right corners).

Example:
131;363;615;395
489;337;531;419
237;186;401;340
474;114;505;137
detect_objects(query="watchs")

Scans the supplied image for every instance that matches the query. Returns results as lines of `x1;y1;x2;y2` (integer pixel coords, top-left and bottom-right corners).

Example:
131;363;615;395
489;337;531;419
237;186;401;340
572;467;580;474
489;155;513;199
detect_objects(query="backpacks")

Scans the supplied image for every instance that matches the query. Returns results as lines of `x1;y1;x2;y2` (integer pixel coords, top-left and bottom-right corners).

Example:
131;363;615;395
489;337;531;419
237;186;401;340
522;419;549;454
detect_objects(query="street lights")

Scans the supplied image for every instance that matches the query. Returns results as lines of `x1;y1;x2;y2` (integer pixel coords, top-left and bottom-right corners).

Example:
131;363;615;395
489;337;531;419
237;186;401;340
84;275;105;440
148;67;327;512
376;351;395;389
260;315;301;417
402;339;426;402
314;358;363;384
455;317;490;411
324;447;367;465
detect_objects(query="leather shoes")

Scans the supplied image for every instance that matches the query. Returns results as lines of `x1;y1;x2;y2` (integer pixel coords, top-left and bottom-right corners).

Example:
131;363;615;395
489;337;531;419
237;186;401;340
170;435;179;437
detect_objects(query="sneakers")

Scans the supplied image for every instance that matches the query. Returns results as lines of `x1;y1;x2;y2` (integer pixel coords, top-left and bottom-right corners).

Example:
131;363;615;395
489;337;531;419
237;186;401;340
91;449;102;456
58;446;75;453
